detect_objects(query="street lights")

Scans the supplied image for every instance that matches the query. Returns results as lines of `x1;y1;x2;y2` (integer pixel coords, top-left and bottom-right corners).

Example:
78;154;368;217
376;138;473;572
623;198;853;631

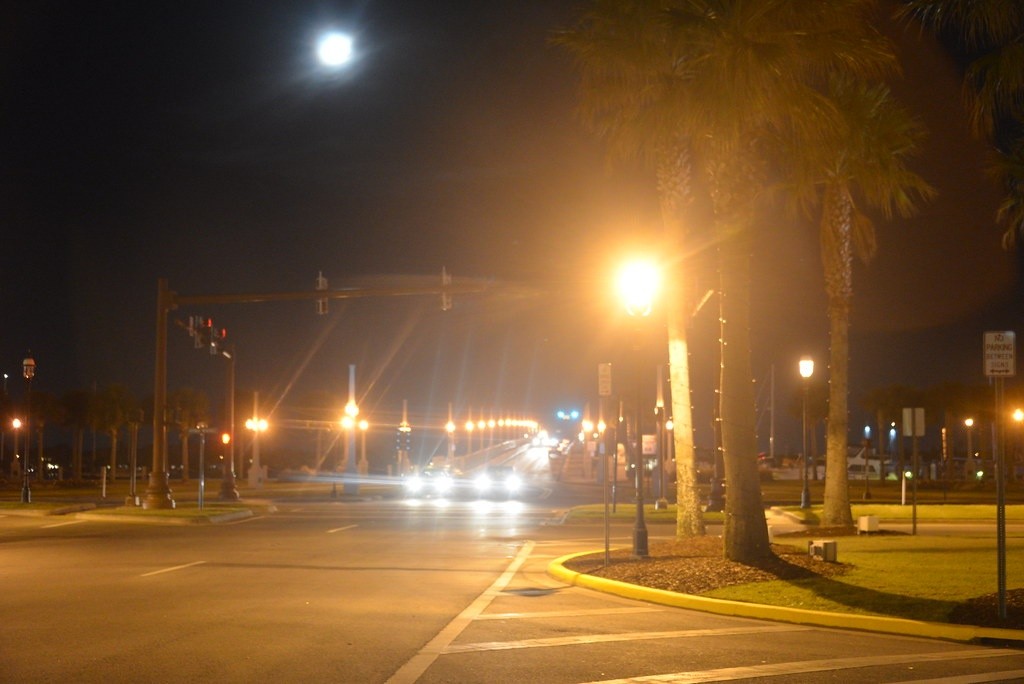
796;354;816;508
